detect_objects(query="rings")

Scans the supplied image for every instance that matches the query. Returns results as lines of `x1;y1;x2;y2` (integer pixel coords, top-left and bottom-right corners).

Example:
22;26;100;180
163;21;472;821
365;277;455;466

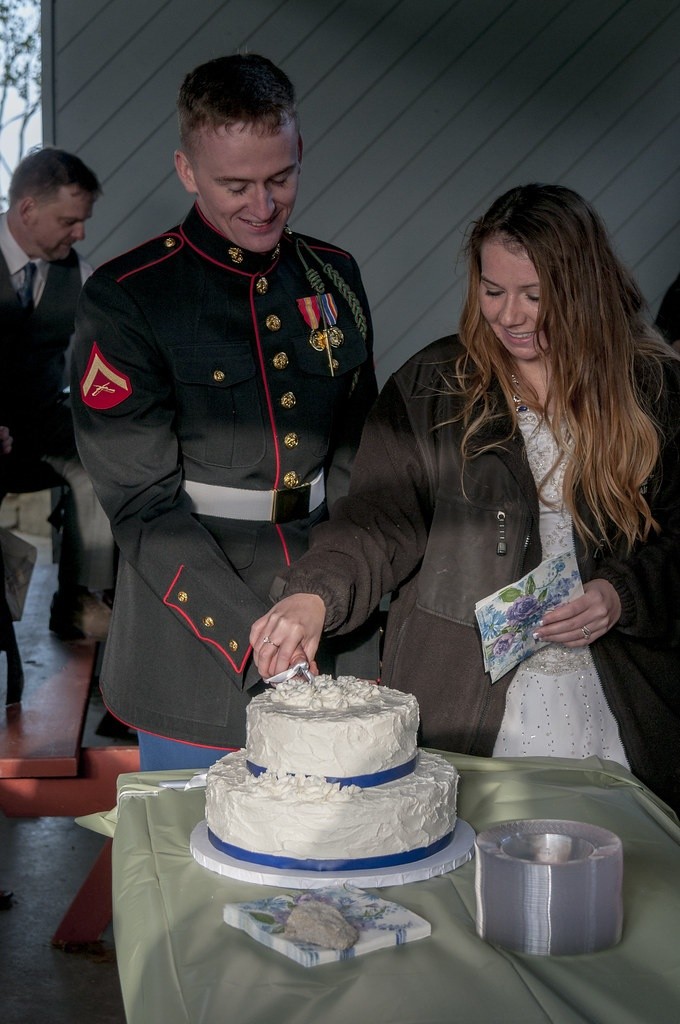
580;625;592;640
263;636;280;648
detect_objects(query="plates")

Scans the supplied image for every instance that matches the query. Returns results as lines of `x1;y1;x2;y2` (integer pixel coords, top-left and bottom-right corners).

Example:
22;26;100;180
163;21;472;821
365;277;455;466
474;818;623;954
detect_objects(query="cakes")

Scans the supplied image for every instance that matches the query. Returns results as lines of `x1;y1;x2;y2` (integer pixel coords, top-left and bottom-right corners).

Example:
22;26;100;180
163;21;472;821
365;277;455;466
204;673;459;871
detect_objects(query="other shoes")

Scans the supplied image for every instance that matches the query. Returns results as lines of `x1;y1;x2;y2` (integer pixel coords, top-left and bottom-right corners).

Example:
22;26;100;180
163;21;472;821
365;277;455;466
49;591;115;639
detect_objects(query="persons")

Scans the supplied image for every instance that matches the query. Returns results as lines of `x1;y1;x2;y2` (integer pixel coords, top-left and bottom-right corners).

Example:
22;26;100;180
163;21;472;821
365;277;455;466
73;52;379;774
247;181;680;828
0;147;112;639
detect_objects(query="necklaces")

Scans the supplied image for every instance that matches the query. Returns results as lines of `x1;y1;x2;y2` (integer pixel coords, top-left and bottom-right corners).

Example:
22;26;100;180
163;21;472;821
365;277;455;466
507;368;530;414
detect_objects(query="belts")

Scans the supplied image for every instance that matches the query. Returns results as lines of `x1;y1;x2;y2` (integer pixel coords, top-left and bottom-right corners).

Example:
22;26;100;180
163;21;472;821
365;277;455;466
179;466;326;525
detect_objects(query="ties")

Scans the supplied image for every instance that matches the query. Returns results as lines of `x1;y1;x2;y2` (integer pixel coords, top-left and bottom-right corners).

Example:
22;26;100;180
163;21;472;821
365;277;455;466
18;262;36;324
